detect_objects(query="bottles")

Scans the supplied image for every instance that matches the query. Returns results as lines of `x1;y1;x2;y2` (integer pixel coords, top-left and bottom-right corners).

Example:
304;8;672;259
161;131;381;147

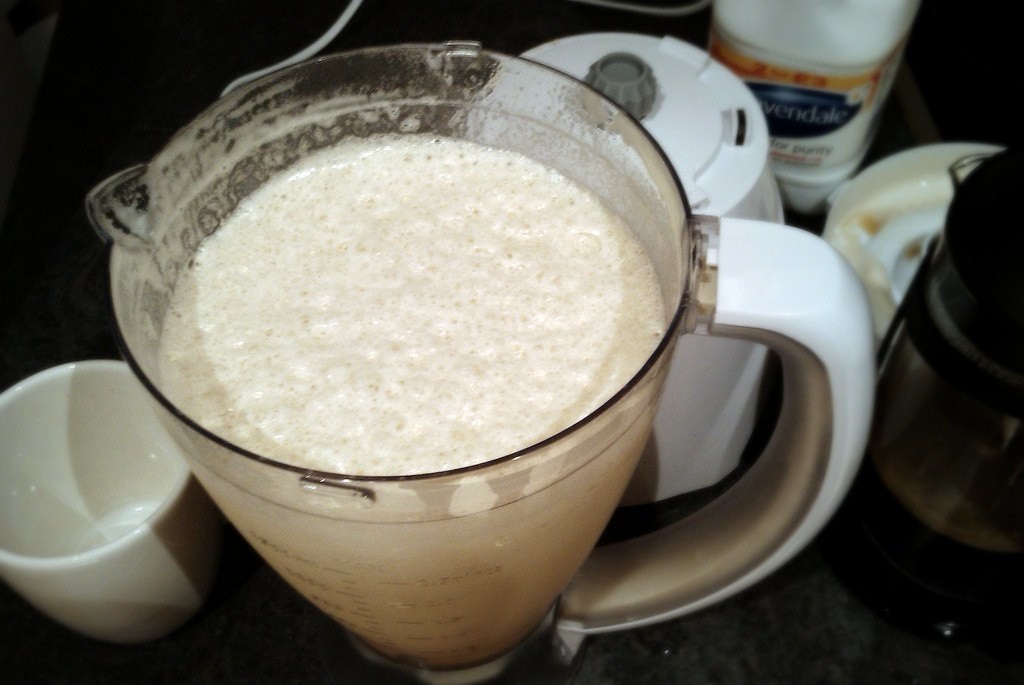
707;0;920;211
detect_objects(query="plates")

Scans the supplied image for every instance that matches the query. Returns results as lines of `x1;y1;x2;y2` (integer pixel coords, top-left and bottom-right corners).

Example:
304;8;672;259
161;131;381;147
824;144;1004;354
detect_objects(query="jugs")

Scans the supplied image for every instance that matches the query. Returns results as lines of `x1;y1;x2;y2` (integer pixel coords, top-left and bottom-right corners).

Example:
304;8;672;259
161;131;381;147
825;143;1023;648
85;45;876;684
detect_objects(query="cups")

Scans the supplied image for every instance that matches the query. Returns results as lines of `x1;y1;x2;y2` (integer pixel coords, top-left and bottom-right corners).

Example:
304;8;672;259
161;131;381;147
0;361;218;645
